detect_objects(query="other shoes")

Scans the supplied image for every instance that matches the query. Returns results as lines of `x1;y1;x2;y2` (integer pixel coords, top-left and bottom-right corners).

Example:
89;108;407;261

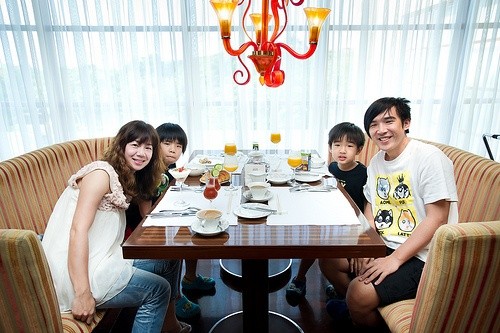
325;284;337;299
286;275;306;297
178;320;192;333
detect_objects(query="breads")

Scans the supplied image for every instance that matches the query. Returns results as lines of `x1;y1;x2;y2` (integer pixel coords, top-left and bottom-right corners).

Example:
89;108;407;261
201;170;230;182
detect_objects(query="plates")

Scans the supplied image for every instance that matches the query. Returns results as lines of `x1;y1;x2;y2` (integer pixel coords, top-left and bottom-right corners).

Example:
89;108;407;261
199;174;229;184
294;172;322;182
266;173;293;185
232;203;273;219
197;157;224;167
191;218;230;237
242;190;274;202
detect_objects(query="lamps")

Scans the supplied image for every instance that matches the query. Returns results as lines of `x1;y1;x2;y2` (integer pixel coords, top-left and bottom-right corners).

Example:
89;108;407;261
211;0;332;87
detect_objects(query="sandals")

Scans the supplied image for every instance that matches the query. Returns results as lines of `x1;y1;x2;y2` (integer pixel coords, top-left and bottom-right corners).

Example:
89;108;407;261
175;295;201;318
181;273;216;289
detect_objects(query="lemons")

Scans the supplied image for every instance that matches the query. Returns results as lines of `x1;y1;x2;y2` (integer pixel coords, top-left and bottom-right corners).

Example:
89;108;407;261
214;163;223;171
211;169;219;177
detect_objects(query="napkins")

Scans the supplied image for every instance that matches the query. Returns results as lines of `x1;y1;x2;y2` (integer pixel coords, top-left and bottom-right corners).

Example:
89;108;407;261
187;155;247;174
266;185;361;225
270;157;333;177
141;186;242;227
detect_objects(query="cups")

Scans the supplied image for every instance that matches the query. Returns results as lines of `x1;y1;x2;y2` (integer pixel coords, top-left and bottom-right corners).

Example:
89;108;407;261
248;182;271;198
225;143;236;155
196;209;222;232
248;151;264;164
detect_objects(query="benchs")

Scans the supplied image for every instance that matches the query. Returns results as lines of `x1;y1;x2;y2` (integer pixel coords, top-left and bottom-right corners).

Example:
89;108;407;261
0;137;115;333
327;133;500;333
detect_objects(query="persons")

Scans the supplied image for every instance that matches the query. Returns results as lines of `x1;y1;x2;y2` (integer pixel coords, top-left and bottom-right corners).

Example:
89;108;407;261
318;97;459;333
125;122;216;318
42;120;193;333
285;122;368;299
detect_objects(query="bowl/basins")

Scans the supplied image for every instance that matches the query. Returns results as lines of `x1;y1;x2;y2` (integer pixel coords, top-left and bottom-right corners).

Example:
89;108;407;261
187;166;204;176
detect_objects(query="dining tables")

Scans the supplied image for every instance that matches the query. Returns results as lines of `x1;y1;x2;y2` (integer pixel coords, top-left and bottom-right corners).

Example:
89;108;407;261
122;149;385;333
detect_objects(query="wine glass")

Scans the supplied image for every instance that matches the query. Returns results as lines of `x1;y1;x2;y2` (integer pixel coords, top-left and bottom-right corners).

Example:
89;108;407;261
203;177;222;208
288;154;302;186
270;132;282;158
168;168;191;207
224;153;239;192
